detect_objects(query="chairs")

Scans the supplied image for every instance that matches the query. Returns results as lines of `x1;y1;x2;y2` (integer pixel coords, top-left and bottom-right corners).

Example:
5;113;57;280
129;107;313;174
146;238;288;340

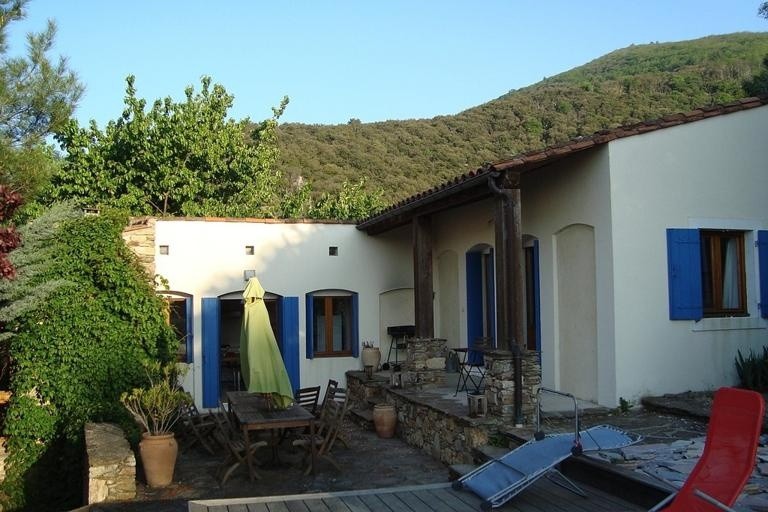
181;392;226;455
288;398;341;476
452;388;645;510
209;410;268;488
315;379;338;419
282;386;320;436
450;335;492;397
648;387;765;512
218;400;255;443
314;387;348;448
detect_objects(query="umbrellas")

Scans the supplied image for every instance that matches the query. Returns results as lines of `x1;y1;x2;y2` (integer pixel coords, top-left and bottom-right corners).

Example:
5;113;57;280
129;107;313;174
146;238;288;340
237;276;299;446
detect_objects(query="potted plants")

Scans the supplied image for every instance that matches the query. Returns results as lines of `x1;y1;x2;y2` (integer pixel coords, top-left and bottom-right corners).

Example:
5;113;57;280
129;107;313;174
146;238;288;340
120;359;194;488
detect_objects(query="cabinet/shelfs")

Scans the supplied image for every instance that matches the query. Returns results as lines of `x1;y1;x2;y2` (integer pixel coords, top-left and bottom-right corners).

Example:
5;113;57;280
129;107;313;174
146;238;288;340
317;314;342;352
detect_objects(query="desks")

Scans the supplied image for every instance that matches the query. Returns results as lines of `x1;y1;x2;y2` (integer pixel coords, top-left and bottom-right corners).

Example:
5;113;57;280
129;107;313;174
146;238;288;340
221;353;240;391
226;392;315;482
